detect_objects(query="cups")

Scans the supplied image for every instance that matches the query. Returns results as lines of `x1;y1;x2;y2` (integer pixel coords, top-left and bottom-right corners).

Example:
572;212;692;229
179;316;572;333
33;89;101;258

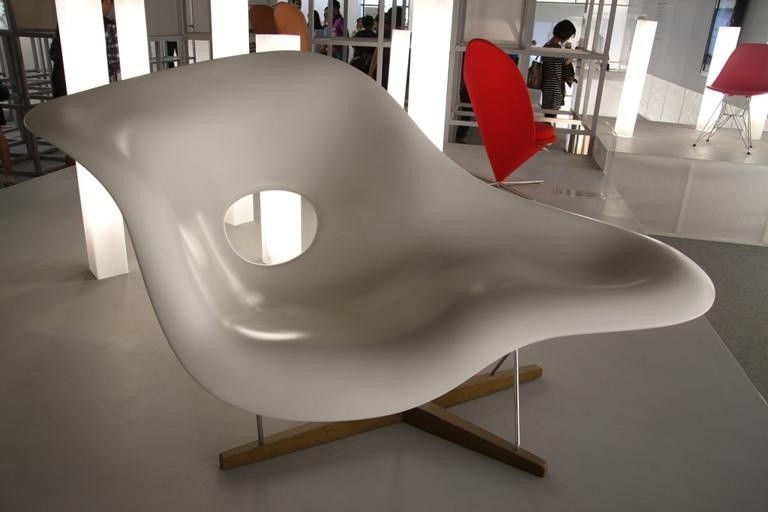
564;42;572;48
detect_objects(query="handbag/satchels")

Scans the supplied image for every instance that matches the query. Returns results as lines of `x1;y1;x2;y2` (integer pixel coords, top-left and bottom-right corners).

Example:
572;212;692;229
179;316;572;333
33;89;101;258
527;62;543;89
0;79;9;102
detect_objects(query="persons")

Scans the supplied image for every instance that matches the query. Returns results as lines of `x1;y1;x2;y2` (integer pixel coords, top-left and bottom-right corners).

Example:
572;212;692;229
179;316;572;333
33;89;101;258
0;79;16;186
542;20;576;147
291;1;402;91
48;0;120;166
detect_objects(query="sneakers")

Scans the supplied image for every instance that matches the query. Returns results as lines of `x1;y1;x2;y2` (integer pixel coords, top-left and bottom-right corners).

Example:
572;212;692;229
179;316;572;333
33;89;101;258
3;177;15;185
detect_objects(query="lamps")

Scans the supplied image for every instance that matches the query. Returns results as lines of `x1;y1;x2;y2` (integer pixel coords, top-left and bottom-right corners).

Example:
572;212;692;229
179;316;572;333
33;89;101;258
692;43;767;154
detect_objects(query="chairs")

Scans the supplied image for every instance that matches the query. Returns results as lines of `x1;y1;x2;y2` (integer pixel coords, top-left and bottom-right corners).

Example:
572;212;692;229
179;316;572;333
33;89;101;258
23;50;717;478
462;38;559;200
